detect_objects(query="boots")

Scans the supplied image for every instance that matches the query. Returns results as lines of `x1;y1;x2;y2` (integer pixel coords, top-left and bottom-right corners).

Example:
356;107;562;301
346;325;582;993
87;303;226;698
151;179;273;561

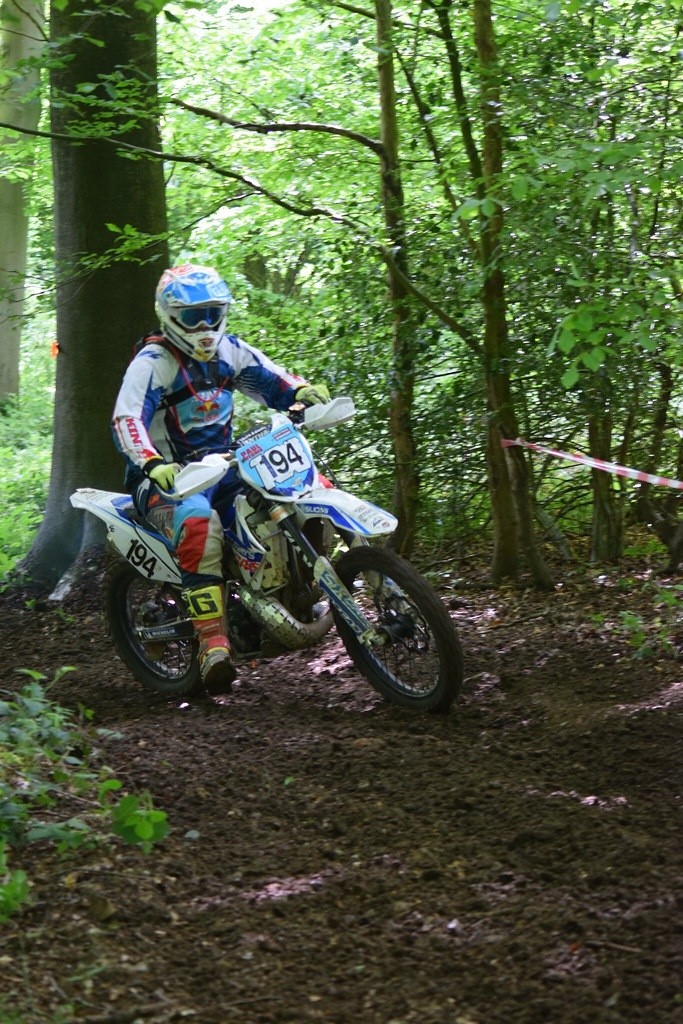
185;585;237;698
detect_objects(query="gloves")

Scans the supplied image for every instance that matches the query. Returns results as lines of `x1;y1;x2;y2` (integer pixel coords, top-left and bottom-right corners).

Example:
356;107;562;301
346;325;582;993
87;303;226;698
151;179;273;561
294;382;332;406
142;459;183;492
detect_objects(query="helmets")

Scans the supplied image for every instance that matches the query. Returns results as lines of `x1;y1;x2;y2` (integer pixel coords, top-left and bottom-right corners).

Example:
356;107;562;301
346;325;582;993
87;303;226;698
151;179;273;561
154;263;233;364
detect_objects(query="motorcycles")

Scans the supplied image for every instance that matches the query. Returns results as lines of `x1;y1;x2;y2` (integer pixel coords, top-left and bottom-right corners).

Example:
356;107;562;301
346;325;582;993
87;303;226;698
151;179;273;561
68;397;465;715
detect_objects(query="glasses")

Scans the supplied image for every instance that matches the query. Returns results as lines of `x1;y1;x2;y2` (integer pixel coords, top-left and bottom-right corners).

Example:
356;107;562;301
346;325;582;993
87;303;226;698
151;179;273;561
168;305;224;331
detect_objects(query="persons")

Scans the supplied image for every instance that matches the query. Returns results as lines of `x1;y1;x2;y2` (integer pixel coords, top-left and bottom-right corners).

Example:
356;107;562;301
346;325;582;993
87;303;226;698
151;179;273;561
108;263;353;698
